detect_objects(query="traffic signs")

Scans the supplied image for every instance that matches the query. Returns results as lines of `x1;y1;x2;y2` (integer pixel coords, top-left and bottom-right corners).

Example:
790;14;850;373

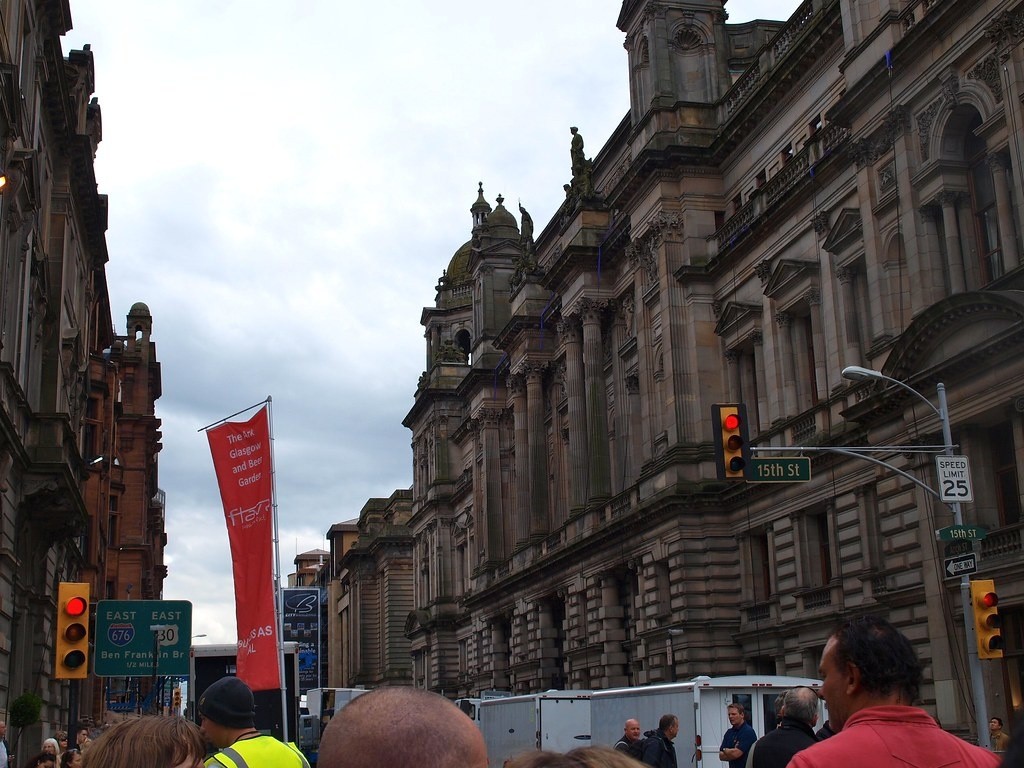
92;599;192;678
943;552;978;581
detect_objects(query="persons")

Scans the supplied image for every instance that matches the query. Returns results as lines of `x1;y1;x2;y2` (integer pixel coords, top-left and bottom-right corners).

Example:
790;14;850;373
570;126;584;160
316;686;490;768
785;609;1002;768
643;713;679;768
199;674;314;768
989;716;1010;751
719;703;758;768
0;720;15;768
503;740;657;768
75;376;85;405
613;717;641;754
746;686;837;768
23;713;110;768
83;711;207;768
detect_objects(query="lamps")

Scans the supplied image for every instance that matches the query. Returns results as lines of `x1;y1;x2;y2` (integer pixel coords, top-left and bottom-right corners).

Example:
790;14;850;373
88;455;103;466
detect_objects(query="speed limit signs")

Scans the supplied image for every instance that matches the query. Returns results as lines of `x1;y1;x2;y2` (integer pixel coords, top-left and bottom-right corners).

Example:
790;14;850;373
935;454;975;504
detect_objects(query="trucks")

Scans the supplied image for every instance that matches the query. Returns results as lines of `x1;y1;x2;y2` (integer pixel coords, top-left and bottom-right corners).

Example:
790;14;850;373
189;639;829;768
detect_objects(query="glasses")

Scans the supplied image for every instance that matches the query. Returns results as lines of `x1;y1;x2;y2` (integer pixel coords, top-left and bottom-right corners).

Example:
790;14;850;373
67;748;77;753
60;737;68;741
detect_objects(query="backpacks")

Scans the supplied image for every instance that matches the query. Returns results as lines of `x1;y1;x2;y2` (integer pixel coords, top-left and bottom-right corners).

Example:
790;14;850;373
627;735;663;761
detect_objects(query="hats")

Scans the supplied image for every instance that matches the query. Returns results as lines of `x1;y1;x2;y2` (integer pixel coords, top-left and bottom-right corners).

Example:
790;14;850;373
197;676;259;729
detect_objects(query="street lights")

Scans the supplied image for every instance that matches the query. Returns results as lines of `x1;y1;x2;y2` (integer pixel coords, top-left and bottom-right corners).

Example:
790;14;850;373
841;365;995;753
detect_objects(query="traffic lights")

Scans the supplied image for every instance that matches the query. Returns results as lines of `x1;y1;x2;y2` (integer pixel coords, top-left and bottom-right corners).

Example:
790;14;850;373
968;578;1005;659
711;402;752;480
55;582;89;679
173;688;181;708
